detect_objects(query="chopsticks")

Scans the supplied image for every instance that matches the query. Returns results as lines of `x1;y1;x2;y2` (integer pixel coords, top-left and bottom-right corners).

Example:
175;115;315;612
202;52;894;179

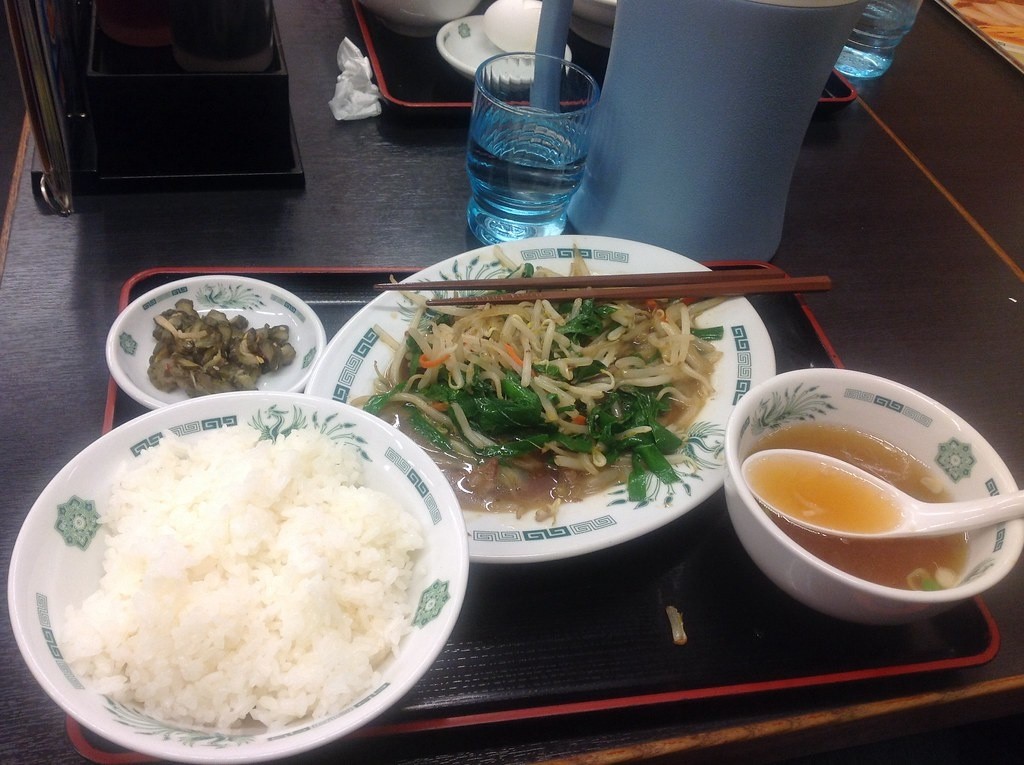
372;269;833;307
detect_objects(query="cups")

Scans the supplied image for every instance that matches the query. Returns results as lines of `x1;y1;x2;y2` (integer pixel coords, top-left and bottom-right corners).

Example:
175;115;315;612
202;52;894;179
834;0;924;78
465;53;603;247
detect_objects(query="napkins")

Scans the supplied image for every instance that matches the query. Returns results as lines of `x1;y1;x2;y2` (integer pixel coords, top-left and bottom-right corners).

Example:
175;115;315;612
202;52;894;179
327;37;382;122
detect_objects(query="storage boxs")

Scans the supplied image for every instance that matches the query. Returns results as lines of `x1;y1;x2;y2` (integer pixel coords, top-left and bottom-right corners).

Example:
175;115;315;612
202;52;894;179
85;0;290;154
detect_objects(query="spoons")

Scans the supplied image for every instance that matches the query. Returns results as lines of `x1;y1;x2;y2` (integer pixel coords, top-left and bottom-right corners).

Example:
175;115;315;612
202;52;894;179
740;448;1024;540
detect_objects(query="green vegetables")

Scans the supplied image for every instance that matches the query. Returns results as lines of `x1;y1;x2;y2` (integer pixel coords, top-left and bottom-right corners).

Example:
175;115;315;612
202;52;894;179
360;262;724;504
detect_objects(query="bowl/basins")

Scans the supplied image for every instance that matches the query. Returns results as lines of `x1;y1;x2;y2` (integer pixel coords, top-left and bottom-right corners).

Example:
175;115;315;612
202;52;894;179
723;368;1024;629
5;390;469;765
358;0;482;38
569;0;618;49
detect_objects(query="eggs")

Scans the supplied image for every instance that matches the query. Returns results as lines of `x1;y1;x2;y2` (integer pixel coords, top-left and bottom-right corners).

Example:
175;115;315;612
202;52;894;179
483;0;542;55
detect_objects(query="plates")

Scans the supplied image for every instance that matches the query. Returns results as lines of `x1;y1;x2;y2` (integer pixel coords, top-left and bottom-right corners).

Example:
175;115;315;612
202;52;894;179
434;14;573;89
105;274;327;413
305;235;777;564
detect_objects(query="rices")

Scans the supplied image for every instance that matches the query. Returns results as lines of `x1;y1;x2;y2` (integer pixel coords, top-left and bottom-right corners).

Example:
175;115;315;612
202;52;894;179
60;424;427;738
